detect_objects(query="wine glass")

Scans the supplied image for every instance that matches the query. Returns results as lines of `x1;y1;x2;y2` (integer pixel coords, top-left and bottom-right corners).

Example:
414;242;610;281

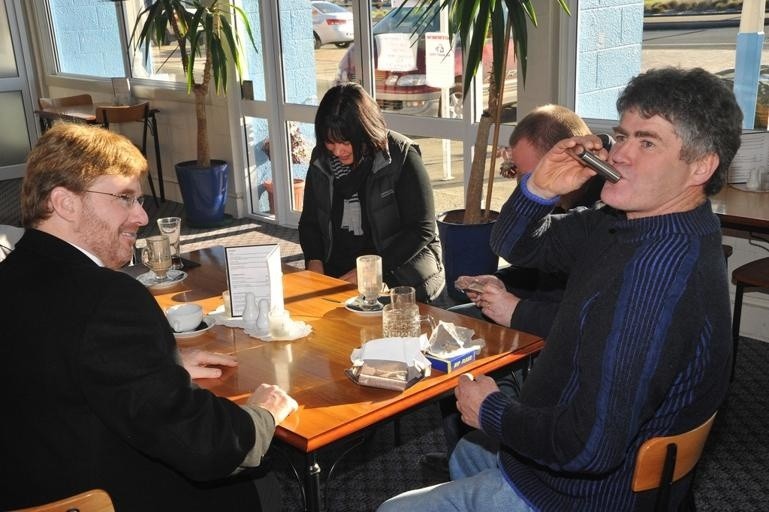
140;235;173;283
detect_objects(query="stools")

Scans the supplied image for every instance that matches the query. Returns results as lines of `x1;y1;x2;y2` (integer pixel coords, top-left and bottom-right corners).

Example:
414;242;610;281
719;244;732;270
728;256;769;383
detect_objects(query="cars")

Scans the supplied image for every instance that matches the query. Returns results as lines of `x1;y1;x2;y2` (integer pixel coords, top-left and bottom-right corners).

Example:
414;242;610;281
309;1;355;50
148;7;203;48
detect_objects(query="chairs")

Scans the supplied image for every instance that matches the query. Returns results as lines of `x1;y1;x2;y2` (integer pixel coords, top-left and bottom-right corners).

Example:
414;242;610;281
629;407;721;512
95;100;160;211
38;94;93;129
9;488;117;512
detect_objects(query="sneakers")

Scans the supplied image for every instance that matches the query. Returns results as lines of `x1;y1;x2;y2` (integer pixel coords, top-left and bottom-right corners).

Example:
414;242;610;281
422;451;450;471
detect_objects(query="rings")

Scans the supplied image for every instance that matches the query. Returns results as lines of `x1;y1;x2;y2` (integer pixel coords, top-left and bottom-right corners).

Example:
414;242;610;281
486;301;492;310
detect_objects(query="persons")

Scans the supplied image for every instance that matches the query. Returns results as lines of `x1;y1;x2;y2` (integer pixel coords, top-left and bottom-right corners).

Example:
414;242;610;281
0;120;299;511
370;64;743;512
299;82;456;309
417;104;625;472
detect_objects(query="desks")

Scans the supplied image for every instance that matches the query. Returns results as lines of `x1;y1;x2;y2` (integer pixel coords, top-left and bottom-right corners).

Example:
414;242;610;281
34;104;160;203
707;181;769;232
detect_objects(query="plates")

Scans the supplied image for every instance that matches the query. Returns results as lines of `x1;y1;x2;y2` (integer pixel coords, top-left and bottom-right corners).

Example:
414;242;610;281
343;295;385;316
170;317;214;338
136;269;187;289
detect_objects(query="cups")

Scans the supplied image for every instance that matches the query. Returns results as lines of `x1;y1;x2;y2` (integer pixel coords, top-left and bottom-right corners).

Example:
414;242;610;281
165;304;202;331
381;302;437;353
389;285;415;309
268;311;290;338
156;218;185;269
221;289;230;317
355;256;383;312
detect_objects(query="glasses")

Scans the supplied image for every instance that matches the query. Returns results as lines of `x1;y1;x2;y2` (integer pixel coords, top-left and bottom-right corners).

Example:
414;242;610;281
71;185;146;209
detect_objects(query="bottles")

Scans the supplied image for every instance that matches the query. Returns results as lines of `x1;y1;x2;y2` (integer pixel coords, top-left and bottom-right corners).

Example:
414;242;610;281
579;151;621;185
241;293;258;323
254;299;270;328
746;167;768;190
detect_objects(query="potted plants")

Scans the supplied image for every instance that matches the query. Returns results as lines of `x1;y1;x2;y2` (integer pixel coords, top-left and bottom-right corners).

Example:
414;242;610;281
391;0;573;303
261;121;306;216
126;0;259;229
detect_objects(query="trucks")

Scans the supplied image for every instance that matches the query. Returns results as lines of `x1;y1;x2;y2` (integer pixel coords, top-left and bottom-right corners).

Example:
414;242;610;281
328;0;519;115
